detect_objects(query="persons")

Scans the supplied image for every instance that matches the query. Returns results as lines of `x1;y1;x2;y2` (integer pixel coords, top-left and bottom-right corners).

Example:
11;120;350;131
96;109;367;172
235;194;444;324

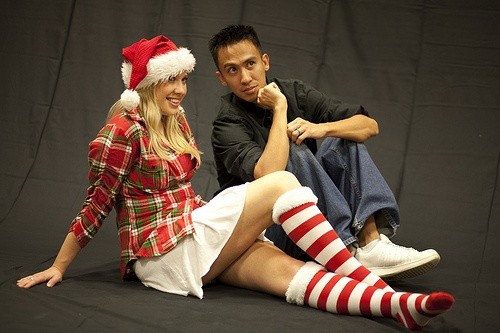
208;24;441;282
17;34;456;332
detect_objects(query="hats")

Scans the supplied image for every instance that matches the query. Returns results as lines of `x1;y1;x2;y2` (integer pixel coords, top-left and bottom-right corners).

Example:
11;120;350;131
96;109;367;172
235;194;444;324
121;35;196;112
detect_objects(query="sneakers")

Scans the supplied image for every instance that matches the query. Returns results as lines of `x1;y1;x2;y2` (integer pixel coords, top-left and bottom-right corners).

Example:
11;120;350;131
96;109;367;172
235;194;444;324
356;233;440;279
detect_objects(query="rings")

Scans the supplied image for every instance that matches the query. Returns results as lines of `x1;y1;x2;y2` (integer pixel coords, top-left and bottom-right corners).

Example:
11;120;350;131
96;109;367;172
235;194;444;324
30;277;34;281
296;128;302;135
